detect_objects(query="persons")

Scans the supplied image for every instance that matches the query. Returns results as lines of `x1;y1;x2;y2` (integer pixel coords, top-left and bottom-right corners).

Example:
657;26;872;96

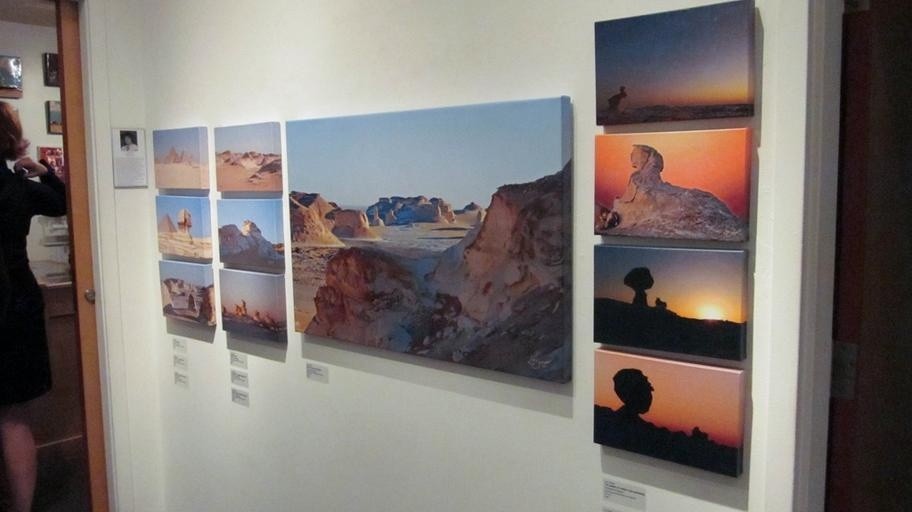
120;133;137;151
0;101;66;512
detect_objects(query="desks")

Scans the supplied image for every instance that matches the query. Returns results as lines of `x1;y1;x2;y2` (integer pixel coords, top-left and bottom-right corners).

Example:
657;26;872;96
10;257;83;484
